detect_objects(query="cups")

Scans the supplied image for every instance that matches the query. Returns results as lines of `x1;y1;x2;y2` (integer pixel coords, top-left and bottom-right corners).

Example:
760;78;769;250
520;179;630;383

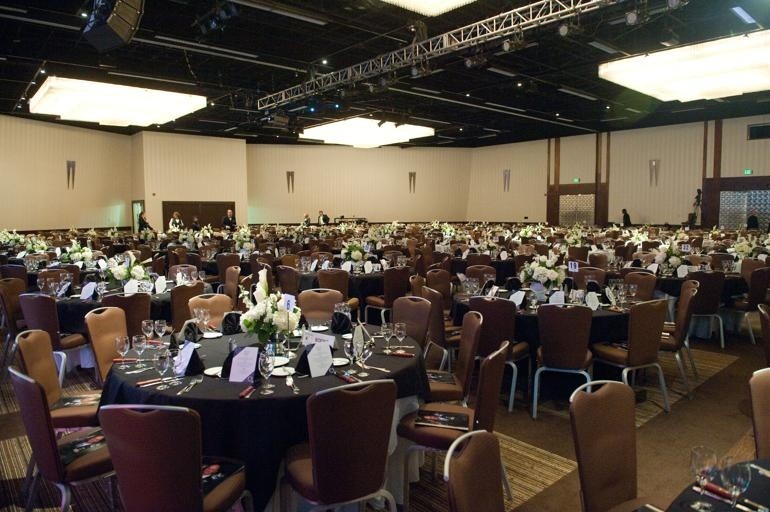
228;335;238;353
334;302;352;321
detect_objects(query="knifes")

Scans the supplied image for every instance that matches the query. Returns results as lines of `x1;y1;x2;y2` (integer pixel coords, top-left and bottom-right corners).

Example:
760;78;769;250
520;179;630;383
112;357;160;361
136;375;184;387
374;345;416;357
207;324;221;333
335;369;363;383
239;379;263;400
141;338;171;346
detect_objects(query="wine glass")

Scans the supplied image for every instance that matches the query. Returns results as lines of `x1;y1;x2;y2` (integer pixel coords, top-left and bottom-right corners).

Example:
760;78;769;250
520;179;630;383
153;352;171;394
114;335;147;371
141;307;212;347
344;340;373;378
36;266;207;300
259;351;277;397
465;273;639;314
294;255;408;276
380;321;407;355
204;246;293;261
689;442;749;512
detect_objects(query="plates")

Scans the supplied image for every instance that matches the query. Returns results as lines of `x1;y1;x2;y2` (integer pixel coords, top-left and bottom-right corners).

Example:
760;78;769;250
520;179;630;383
204;366;224;377
332;356;349;367
311;325;329;332
266;354;296;378
342;333;353;340
202;330;222;339
178;343;202;349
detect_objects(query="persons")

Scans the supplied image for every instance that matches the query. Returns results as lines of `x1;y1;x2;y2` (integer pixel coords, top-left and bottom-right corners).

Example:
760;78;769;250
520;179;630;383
169;210;184;231
302;213;311;227
316;210;330;225
136;212;152;231
621;208;633;227
747;209;758;230
222;209;236;232
191;216;202;230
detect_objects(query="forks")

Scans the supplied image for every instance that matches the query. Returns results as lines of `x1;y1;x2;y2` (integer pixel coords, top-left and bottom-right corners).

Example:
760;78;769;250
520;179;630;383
355;360;392;374
